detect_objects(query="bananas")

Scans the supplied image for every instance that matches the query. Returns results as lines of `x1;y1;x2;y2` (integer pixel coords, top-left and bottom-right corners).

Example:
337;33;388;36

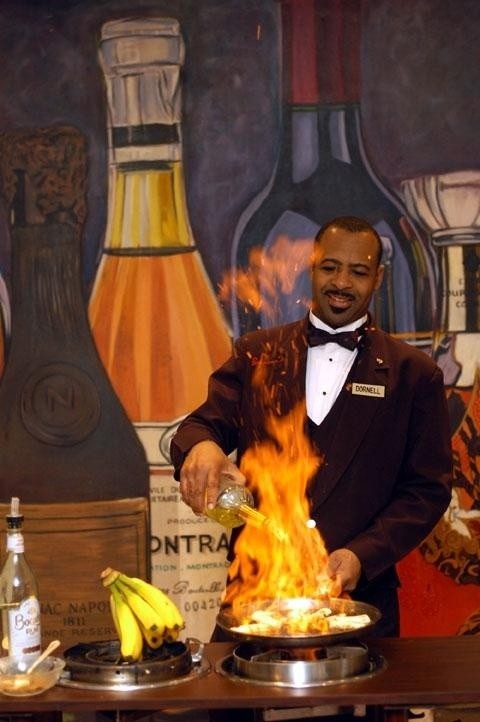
98;567;186;664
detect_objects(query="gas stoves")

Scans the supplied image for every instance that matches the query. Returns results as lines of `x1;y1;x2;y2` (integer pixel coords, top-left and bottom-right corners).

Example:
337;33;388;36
61;639;381;692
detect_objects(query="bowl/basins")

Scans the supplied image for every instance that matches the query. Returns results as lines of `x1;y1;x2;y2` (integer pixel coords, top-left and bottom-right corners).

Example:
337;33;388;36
0;655;65;698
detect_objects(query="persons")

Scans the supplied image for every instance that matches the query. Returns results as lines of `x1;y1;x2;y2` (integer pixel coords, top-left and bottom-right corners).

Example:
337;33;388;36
169;218;455;640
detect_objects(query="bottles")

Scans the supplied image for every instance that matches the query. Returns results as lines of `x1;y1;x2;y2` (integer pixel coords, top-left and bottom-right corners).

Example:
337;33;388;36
0;495;47;655
157;419;270;527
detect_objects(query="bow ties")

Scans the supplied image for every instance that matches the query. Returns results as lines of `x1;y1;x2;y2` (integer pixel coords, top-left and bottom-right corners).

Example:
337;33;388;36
304;327;359;351
